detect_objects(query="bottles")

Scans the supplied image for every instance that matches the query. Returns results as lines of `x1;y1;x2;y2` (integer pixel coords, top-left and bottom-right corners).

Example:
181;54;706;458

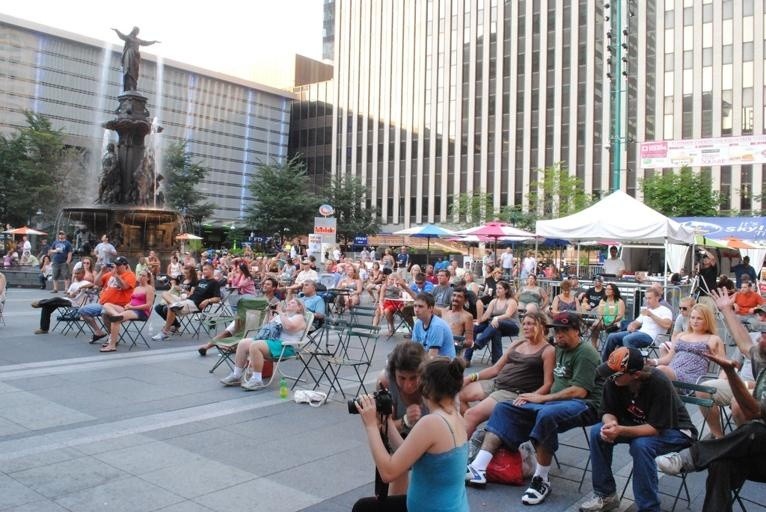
279;377;287;401
148;323;153;339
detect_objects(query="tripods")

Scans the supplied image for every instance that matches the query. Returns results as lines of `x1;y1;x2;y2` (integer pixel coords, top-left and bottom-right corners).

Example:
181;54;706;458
689;258;711;303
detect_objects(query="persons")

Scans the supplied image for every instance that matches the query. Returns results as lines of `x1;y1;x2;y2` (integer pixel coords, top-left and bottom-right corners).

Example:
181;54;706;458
457;314;603;506
580;346;699;511
0;186;764;416
655;322;765;511
354;355;474;512
456;314;557;439
695;380;757;442
368;340;458;497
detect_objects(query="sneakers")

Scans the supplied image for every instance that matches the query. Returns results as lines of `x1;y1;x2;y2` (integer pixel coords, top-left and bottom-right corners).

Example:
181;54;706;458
35;328;48;333
195;314;205;320
240;376;265;390
152;330;169;342
169;301;184;312
522;475;551;505
465;464;487;486
654;451;684;475
32;300;41;308
581;490;619;512
219;373;249;385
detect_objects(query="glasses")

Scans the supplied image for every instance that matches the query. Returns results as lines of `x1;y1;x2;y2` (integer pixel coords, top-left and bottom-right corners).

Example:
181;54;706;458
678;306;687;311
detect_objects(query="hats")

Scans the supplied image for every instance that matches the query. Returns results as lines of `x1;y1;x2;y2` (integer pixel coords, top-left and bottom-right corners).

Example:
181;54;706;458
754;304;766;315
544;311;580;328
113;256;129;266
595;347;644;382
751;321;766;333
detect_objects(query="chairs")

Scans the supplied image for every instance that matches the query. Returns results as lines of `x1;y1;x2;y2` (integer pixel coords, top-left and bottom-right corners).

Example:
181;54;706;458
0;279;10;328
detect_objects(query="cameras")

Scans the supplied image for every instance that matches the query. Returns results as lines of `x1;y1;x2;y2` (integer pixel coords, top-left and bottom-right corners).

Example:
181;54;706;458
269;303;276;310
695;248;706;256
348;389;393;415
106;263;112;268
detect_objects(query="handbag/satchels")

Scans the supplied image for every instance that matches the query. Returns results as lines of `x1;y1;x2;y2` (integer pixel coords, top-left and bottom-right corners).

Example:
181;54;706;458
294;389;327;408
255;320;282;340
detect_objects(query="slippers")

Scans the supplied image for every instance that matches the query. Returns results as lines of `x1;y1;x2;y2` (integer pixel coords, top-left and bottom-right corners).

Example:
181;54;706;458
103;341;118;347
100;346;117;352
109;316;124;322
89;334;107;344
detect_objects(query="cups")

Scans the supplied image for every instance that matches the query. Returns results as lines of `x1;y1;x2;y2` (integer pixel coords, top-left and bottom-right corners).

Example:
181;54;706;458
342;279;347;290
641;351;649;364
402;292;408;300
609;306;615;315
640;306;647;316
181;294;187;300
488;288;493;297
581;302;588;314
599;302;606;315
